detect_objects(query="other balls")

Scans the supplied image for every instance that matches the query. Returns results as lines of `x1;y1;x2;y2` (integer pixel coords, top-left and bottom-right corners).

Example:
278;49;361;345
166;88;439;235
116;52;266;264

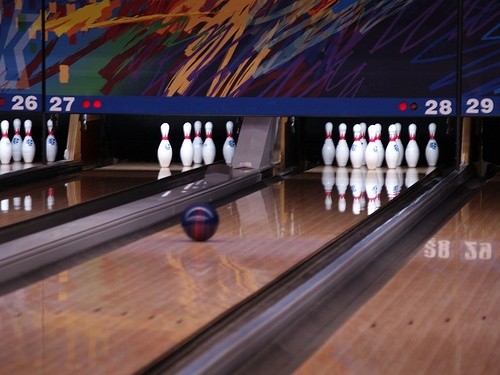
178;201;221;242
